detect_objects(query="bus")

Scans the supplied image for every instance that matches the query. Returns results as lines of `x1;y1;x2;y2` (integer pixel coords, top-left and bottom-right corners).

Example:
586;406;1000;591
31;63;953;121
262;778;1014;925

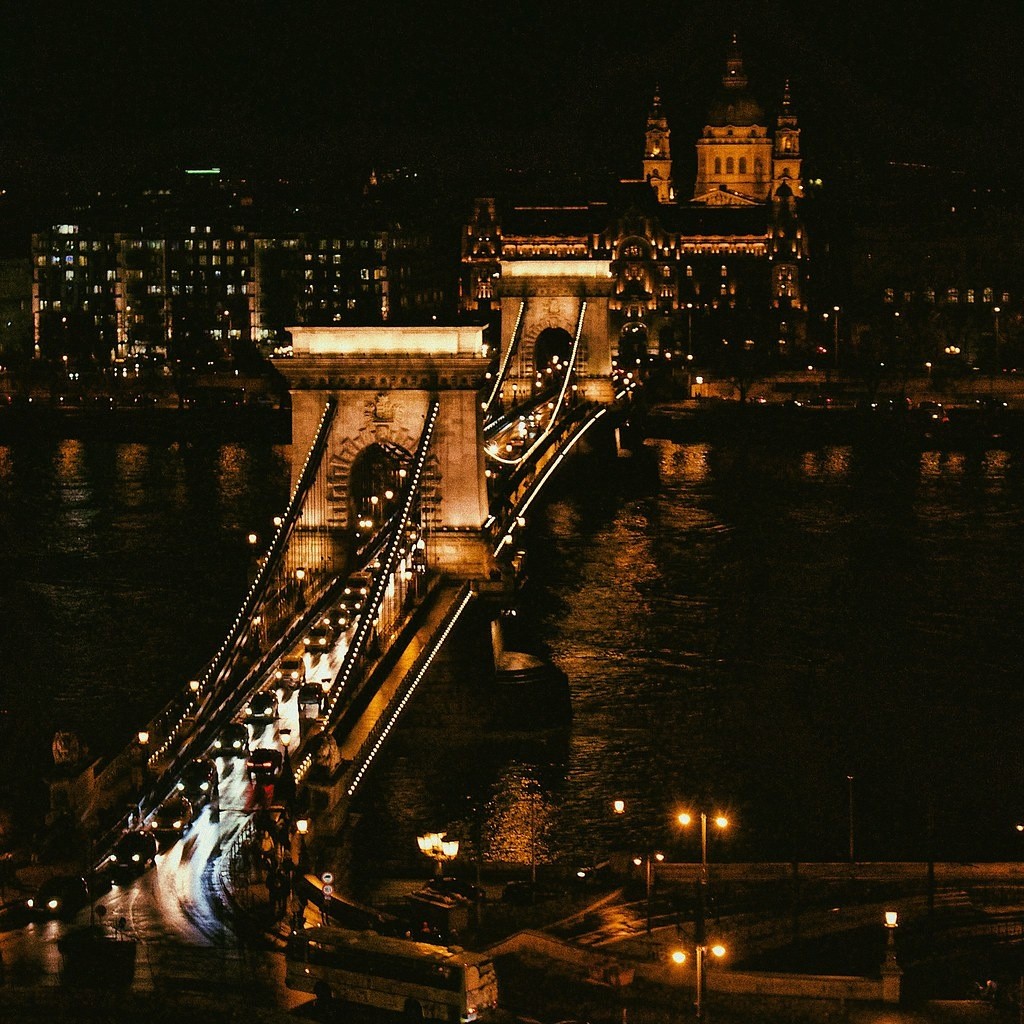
285;921;500;1024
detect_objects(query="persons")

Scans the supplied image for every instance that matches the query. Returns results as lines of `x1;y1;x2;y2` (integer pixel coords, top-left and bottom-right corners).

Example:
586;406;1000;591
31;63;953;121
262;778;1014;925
242;818;314;918
404;922;458;946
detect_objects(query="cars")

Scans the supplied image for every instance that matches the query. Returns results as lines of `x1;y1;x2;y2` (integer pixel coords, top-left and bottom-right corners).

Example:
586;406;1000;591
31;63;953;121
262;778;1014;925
242;748;284;781
178;761;219;801
24;875;92;922
150;796;195;836
481;395;568;453
109;829;157;875
213;552;428;755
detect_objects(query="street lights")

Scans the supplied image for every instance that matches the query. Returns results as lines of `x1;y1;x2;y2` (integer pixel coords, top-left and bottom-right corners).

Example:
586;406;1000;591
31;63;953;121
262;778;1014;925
676;809;729;916
881;909;900;971
673;942;727;1023
633;849;665;931
296;814;316;874
417;828;460;892
